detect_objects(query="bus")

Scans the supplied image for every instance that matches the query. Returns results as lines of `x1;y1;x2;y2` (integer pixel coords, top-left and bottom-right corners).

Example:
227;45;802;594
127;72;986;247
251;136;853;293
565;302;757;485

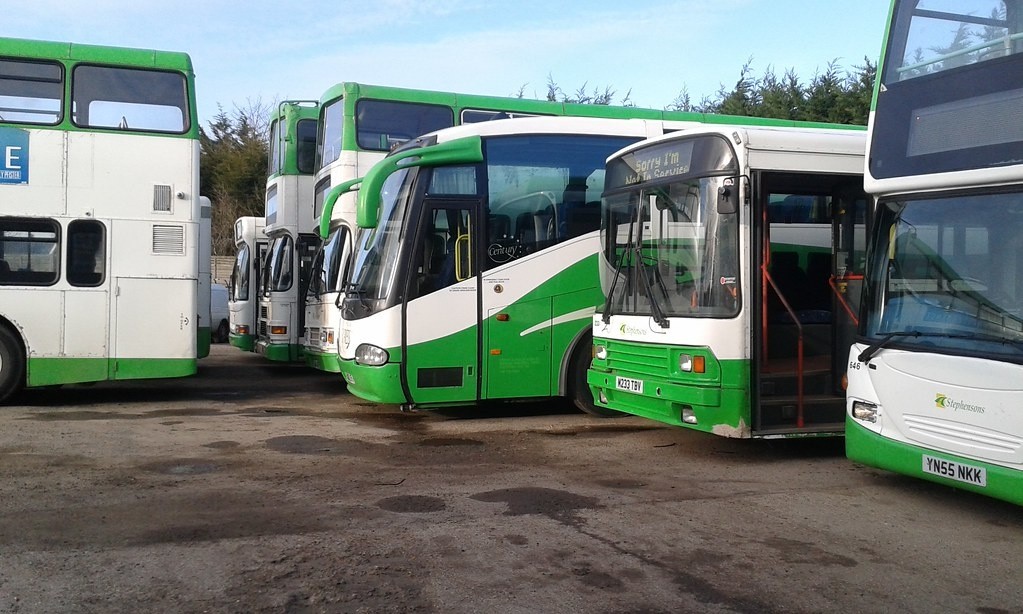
585;123;994;441
225;79;994;373
0;36;212;404
845;0;1023;507
319;116;875;418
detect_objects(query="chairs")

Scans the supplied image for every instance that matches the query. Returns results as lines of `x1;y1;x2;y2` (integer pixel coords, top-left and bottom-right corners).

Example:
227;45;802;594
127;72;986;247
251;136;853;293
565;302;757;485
770;251;834;308
119;116;128;129
770;195;821;222
489;200;603;262
0;259;11;284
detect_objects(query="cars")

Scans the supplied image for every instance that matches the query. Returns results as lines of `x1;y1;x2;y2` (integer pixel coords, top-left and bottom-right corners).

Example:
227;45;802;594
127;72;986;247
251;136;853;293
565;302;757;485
209;281;229;344
